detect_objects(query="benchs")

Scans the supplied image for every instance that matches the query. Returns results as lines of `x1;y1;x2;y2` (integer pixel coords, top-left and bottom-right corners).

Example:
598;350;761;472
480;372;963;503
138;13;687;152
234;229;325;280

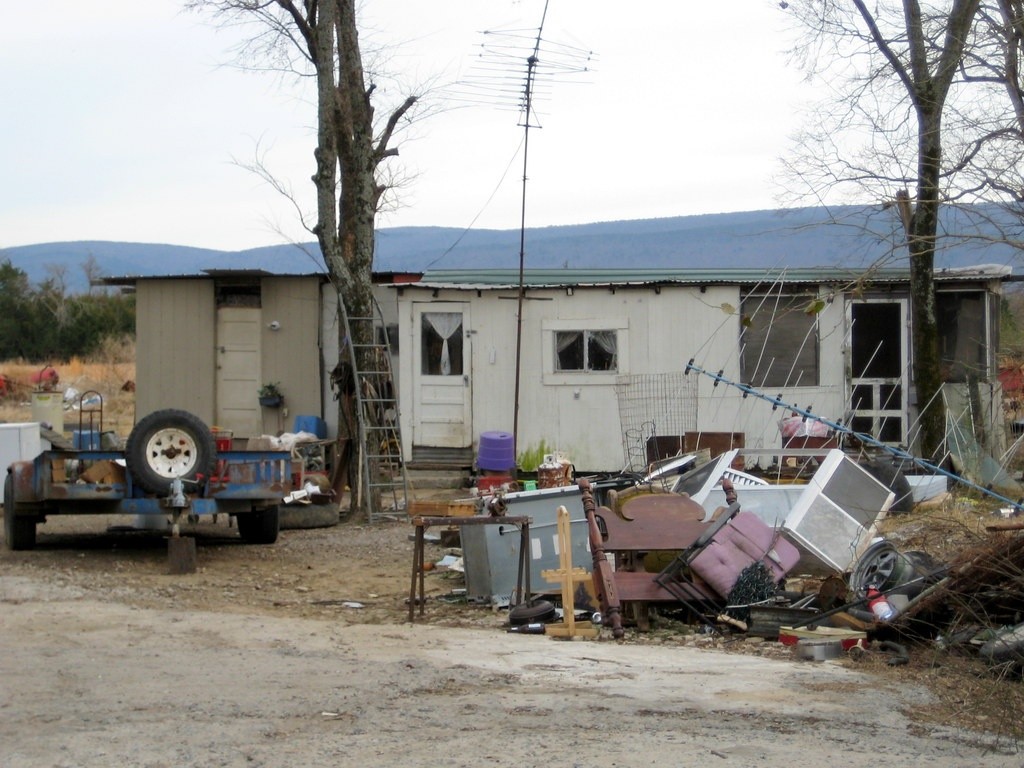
408;516;531;626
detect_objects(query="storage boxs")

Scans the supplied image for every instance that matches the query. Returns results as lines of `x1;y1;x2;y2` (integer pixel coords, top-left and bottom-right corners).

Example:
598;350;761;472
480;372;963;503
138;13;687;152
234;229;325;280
781;436;837;466
294;415;327;439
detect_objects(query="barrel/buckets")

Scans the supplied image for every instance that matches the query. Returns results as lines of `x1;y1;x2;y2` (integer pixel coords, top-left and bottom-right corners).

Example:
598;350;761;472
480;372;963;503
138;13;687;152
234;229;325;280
475;431;516;470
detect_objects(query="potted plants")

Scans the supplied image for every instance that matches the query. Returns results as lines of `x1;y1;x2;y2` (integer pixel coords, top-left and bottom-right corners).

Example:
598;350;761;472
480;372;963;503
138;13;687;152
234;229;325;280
258;381;284;408
